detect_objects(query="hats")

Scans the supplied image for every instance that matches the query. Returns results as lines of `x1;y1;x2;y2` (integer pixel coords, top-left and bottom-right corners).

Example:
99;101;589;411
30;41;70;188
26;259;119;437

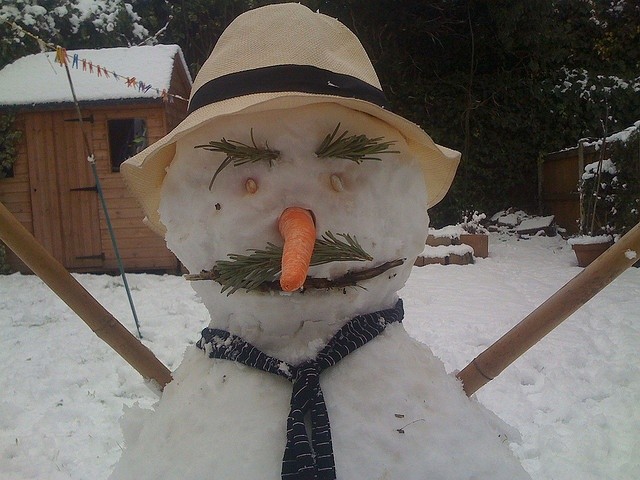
118;2;462;239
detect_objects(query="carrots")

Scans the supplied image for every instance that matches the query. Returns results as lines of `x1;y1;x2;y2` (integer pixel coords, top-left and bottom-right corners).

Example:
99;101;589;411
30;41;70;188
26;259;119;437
277;207;317;292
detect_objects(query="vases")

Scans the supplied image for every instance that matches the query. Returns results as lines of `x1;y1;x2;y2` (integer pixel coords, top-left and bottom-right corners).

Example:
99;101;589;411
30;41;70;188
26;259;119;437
571;242;612;268
